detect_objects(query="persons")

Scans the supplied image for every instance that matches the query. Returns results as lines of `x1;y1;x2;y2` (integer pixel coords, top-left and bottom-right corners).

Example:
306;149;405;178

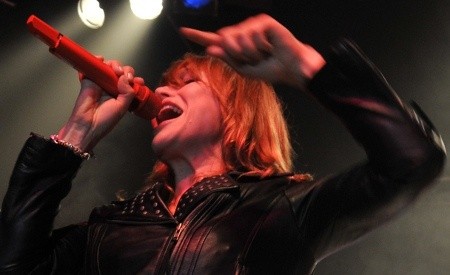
0;16;447;275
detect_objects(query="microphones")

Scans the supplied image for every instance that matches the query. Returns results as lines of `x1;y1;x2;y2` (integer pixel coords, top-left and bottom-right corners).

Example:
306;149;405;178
27;14;163;120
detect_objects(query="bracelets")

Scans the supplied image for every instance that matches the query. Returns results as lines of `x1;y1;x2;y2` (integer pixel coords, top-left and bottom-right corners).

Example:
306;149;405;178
49;133;96;161
